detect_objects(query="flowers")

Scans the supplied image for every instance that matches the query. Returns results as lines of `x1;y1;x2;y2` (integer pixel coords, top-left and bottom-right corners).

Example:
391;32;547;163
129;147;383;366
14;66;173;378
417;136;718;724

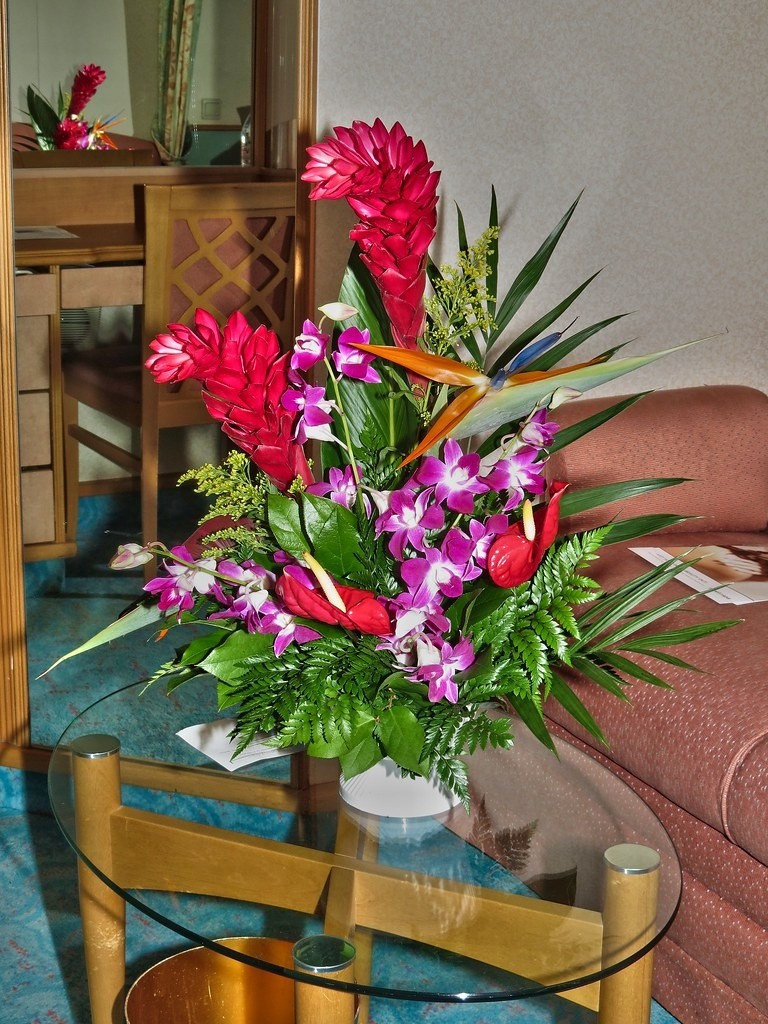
34;116;744;817
14;64;126;150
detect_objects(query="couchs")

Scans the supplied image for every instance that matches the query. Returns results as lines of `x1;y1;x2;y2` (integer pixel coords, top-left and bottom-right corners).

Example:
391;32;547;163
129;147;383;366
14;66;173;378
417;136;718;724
442;385;768;1024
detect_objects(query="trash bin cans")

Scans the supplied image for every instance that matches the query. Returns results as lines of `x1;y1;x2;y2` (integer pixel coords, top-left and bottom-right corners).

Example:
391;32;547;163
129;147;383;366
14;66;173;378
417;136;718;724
122;932;361;1024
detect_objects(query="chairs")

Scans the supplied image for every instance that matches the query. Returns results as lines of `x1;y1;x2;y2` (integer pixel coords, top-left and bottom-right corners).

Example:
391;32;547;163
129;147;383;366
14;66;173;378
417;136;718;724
68;181;293;582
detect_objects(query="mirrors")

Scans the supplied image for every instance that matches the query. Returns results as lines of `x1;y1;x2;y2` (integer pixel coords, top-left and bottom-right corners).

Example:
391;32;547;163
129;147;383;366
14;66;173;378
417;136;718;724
7;0;266;168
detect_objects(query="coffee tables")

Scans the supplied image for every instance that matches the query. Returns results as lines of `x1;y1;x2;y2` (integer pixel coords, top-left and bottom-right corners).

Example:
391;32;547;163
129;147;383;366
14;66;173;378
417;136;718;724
43;668;681;1022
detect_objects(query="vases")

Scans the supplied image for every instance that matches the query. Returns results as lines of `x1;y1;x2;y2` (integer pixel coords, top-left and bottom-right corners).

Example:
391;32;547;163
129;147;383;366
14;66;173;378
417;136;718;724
338;707;476;817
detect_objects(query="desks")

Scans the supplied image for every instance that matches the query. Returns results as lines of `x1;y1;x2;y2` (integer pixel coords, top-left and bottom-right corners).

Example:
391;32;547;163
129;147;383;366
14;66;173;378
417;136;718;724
13;225;147;561
